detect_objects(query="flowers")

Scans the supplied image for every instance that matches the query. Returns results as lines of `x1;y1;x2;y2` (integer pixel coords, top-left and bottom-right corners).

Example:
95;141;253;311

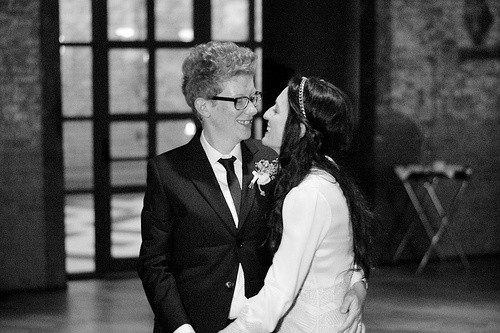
248;158;279;196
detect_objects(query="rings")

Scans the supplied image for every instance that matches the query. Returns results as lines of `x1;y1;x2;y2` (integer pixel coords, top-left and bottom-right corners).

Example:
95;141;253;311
357;324;362;328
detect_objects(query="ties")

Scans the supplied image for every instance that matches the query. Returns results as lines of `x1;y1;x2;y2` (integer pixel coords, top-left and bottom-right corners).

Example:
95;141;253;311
218;158;242;222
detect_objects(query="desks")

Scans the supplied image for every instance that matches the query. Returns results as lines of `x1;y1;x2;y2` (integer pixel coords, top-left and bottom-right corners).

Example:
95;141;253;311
386;170;475;279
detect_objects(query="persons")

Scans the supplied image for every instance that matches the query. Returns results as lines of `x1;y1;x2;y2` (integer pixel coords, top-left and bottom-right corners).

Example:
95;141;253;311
219;75;371;333
137;41;370;333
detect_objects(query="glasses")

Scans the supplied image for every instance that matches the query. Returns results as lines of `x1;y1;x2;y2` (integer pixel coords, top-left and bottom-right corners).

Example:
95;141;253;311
204;89;263;110
